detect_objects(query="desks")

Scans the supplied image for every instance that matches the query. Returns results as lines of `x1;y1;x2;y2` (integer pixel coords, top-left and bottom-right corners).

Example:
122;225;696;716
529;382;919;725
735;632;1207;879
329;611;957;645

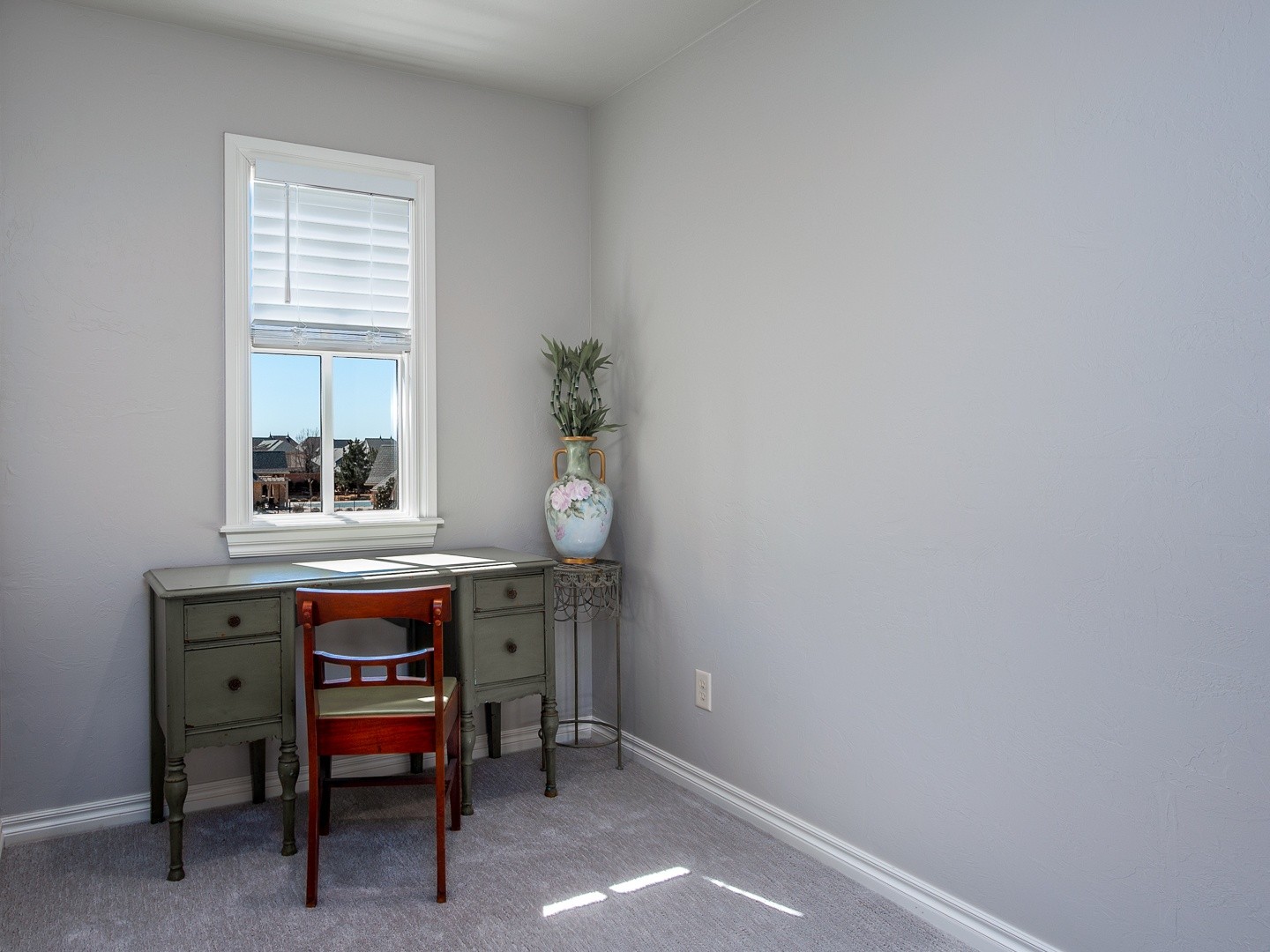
551;560;622;772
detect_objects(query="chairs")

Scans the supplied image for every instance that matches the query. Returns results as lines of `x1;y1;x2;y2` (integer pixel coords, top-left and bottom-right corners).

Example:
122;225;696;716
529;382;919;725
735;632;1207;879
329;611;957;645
295;586;460;908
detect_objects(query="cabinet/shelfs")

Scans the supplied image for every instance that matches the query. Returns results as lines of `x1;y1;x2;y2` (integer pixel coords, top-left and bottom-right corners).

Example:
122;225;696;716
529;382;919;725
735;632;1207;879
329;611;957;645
143;546;559;882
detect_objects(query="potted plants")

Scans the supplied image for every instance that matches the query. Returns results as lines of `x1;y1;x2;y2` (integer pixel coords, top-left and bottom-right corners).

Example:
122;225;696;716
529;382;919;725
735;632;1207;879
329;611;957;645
541;333;627;565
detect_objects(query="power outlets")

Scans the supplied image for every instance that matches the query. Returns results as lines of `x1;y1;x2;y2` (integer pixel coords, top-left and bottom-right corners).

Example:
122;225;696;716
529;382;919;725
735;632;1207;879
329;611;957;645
696;669;711;712
